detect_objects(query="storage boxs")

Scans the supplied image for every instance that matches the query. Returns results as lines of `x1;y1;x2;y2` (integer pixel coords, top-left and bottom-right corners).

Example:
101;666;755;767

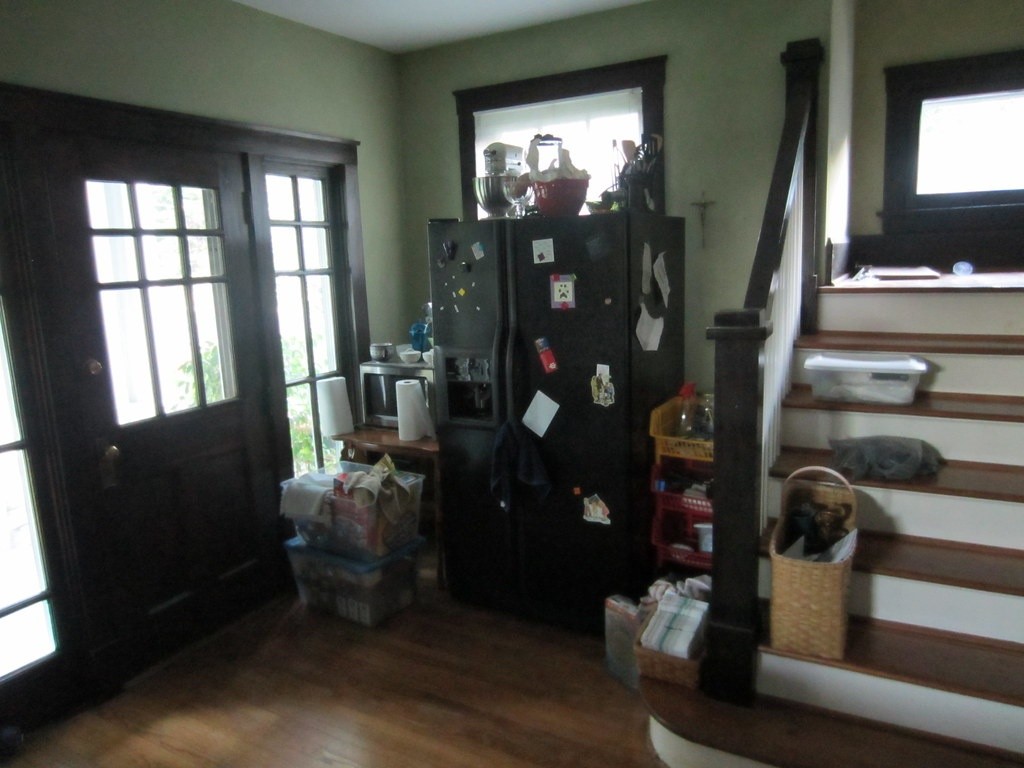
648;394;714;464
279;461;426;564
803;352;928;406
651;550;712;598
650;508;714;563
632;603;707;690
605;594;639;693
649;456;716;518
284;535;426;627
767;465;859;663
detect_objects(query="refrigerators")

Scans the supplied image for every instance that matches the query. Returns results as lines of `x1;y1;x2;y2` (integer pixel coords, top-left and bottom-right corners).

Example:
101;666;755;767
424;210;687;632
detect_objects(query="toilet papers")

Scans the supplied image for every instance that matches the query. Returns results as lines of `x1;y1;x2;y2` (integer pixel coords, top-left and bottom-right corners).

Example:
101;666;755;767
396;380;436;440
316;377;354;436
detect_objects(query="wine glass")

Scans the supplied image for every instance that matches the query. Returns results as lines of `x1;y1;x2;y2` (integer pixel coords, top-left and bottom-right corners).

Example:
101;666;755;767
501;181;534;218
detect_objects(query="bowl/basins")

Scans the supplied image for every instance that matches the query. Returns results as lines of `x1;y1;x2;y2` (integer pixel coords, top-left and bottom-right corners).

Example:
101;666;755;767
370;343;393;361
534;179;588;215
400;351;421;362
472;176;518;217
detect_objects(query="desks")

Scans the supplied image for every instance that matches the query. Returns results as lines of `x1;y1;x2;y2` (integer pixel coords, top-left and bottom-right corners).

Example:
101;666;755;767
332;430;448;578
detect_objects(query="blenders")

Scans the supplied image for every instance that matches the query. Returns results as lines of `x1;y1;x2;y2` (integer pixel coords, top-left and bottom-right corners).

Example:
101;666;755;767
472;142;527;219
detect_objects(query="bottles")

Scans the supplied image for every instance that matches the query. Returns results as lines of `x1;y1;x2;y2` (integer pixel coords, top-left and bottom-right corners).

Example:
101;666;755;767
676;386;693;436
693;391;714;440
